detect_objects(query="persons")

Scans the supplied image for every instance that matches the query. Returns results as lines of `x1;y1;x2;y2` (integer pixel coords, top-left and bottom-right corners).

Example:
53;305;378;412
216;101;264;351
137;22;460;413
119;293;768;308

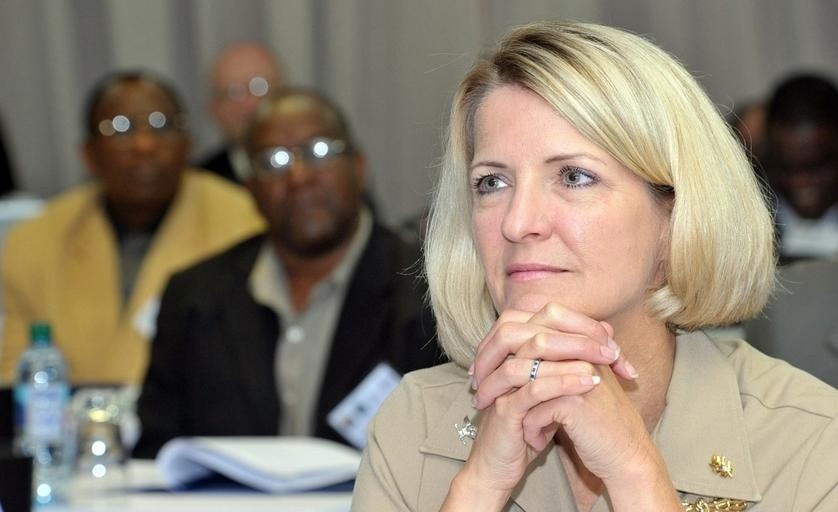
762;67;837;264
348;15;838;511
190;44;290;182
1;65;270;397
127;87;450;460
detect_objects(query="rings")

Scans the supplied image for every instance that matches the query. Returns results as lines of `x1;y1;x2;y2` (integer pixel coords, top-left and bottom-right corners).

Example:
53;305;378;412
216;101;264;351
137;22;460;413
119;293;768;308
525;357;546;385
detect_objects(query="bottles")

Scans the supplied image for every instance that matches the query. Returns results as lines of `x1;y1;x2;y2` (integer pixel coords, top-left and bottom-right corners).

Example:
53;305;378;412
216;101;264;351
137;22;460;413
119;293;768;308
14;319;71;502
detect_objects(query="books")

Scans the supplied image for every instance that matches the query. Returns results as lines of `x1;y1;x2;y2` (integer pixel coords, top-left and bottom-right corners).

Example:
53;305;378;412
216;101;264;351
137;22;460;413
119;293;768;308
55;430;369;495
